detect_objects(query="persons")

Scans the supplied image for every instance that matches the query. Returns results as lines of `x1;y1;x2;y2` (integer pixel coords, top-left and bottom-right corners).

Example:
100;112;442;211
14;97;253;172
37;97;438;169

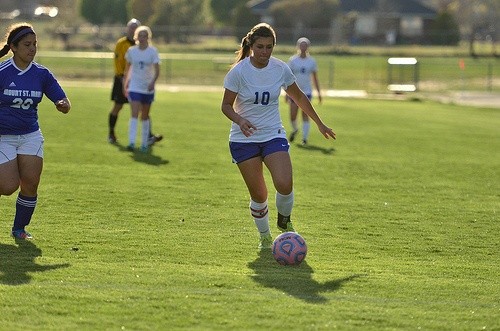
107;18;162;146
222;23;336;248
0;26;70;240
121;26;160;152
285;37;322;144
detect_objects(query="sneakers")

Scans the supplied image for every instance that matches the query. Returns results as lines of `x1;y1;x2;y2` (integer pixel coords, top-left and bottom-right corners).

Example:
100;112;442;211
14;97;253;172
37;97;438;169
149;135;162;146
258;235;274;248
277;220;298;234
10;226;33;239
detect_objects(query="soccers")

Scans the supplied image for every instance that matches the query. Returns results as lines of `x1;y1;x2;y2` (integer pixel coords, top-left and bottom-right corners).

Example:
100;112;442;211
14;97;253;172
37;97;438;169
272;232;308;266
